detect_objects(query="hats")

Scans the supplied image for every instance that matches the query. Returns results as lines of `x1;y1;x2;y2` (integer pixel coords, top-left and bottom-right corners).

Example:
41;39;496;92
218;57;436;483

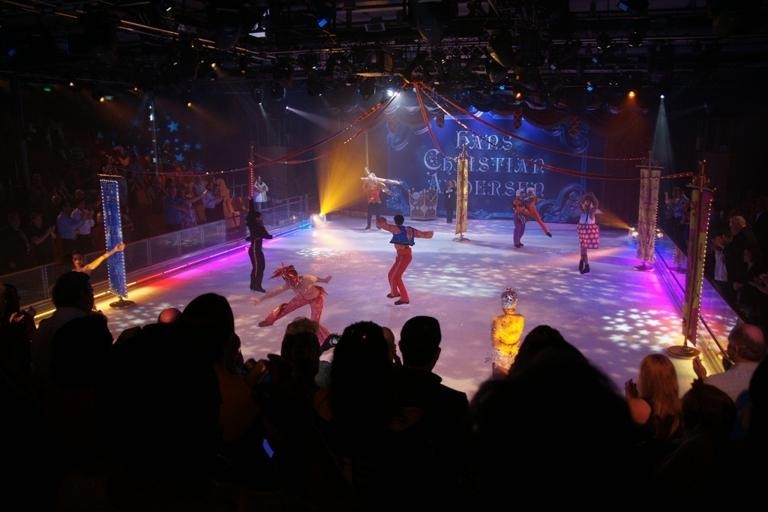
527;188;536;195
516;189;526;197
582;191;599;211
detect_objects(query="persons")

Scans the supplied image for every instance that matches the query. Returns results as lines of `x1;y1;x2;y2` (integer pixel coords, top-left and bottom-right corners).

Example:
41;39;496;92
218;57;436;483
576;193;598;273
30;212;57;265
360;167;403;197
512;189;530;248
727;215;758;276
73;201;95;251
252;265;331;328
492;290;524;376
67;243;126;278
364;186;382;229
732;249;757;319
57;201;88;261
253;174;267;212
97;144;239;246
0;276;630;512
625;354;679;423
444;184;455;224
245;199;274;292
524;187;552;238
376;215;433;305
659;184;692;241
692;324;766;397
707;230;727;290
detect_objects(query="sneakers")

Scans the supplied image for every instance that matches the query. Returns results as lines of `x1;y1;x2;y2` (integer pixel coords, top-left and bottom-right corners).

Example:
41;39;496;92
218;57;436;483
519;243;523;247
545;232;552;237
578;261;584;272
250;284;254;291
387;293;401;298
253;286;266;293
259;320;273;328
582;264;590;274
514;245;520;248
394;300;410;306
